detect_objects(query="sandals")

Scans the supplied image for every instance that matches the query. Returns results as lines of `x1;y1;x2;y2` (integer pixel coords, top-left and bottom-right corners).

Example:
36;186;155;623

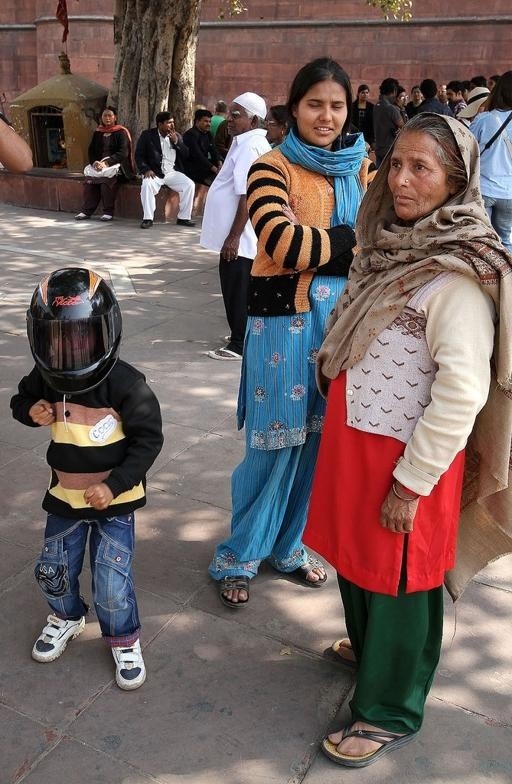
219;575;250;608
289;554;327;586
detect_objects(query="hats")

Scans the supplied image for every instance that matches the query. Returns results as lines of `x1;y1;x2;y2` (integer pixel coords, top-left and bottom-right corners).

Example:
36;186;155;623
457;86;491;118
233;92;267;120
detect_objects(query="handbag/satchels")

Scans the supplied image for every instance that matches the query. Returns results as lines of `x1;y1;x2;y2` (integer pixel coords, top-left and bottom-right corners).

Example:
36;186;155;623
84;157;121;179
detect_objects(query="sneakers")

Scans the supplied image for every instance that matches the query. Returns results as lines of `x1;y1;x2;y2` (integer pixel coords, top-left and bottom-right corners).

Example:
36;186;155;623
111;639;146;690
32;613;85;663
141;220;153;228
75;213;88;219
101;215;113;221
177;219;196;225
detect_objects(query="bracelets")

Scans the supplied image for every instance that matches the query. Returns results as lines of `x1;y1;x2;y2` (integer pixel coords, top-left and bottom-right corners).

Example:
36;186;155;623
392;482;420;503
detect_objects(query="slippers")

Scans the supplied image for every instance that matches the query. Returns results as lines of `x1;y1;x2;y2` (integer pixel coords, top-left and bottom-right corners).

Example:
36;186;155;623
332;638;357;666
209;347;244;361
321;720;420;767
224;336;232;343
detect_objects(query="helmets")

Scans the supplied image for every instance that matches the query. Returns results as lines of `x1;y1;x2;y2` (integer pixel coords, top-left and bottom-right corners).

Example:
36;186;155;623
27;268;122;393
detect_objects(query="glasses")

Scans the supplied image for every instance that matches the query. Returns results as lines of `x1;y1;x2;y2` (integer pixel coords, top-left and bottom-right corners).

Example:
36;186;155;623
400;95;408;100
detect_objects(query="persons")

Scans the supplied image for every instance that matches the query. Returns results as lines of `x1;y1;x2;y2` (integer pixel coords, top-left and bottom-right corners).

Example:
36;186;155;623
9;267;163;691
264;105;289;146
467;69;512;254
76;100;233;228
206;56;378;611
0;112;34;176
199;91;274;361
350;70;499;163
300;109;512;772
